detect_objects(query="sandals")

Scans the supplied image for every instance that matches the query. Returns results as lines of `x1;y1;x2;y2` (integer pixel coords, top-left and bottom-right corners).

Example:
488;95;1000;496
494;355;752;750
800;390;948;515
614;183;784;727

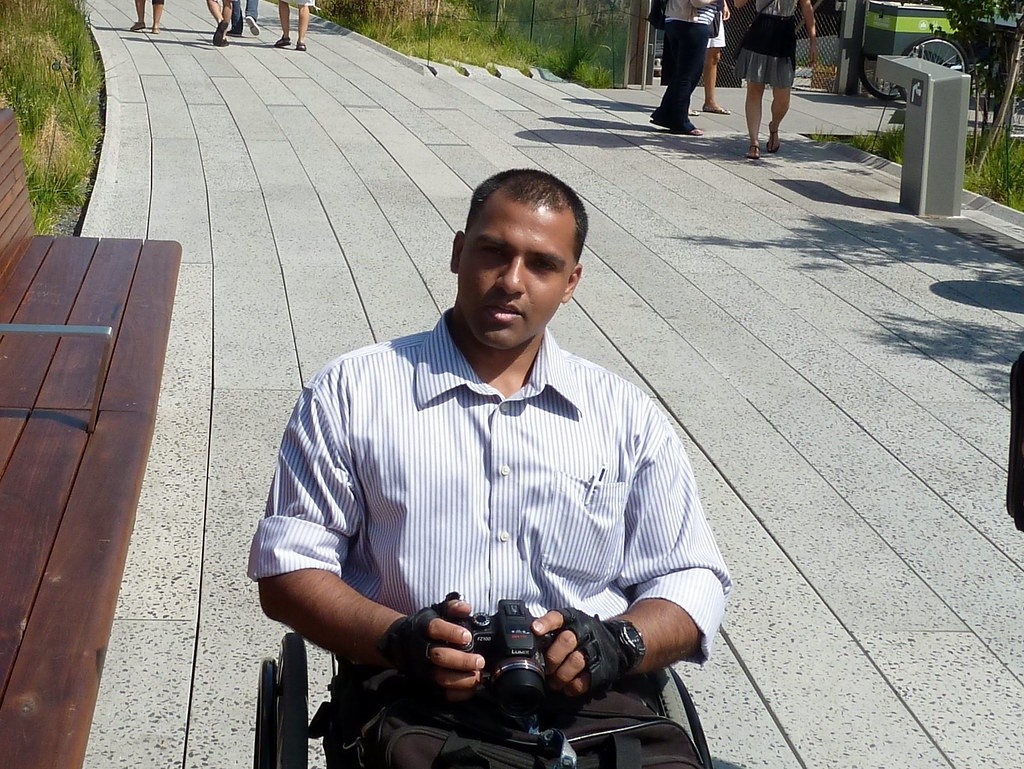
747;145;760;159
274;35;291;47
296;41;306;51
766;122;780;153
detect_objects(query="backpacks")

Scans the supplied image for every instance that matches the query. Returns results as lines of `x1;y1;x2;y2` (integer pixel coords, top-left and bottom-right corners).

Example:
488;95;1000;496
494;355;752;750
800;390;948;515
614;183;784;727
647;0;669;30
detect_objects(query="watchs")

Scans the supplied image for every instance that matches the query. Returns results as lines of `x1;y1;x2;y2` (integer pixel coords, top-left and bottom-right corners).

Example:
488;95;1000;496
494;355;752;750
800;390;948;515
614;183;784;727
604;618;647;673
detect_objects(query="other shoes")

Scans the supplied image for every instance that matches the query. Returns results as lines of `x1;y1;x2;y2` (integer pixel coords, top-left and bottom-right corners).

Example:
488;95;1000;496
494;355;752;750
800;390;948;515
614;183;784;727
703;104;731;115
688;110;700;116
152;28;160;34
130;22;146;31
226;30;242;37
213;19;229;47
649;114;670;128
246;16;260;36
670;127;703;136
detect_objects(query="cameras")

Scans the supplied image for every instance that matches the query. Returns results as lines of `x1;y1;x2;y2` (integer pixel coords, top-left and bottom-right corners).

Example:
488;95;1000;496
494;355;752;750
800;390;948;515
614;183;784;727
453;599;561;718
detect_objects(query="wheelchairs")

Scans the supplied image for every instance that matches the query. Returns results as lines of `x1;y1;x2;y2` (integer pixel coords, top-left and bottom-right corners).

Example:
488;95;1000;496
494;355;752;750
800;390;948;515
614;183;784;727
255;633;715;769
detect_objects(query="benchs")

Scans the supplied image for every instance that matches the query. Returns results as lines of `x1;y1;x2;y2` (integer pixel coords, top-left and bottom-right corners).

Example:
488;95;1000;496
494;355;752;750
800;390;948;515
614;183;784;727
0;106;183;769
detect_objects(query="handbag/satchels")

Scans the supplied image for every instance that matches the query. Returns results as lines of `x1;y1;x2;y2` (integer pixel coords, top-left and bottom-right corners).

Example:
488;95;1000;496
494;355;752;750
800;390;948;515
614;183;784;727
732;36;745;60
342;650;705;769
707;11;720;38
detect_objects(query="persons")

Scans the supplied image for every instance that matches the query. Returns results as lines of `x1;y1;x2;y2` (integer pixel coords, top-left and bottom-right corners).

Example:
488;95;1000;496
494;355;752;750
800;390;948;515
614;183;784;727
226;0;260;37
688;0;731;116
734;0;818;159
650;0;724;136
275;0;315;51
246;165;732;769
130;0;165;33
206;0;233;47
